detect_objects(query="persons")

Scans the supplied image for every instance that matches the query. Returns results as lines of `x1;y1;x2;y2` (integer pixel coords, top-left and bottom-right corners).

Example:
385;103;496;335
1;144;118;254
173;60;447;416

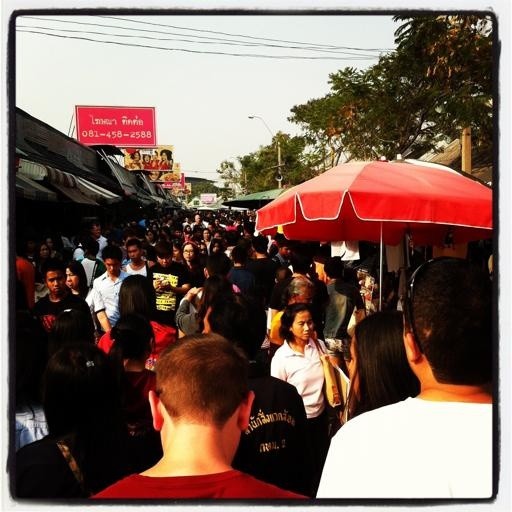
124;148;191;195
11;208;421;499
317;257;493;498
88;334;312;499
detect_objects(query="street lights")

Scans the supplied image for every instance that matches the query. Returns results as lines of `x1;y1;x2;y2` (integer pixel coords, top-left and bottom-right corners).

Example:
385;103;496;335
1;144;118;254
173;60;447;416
247;113;283;190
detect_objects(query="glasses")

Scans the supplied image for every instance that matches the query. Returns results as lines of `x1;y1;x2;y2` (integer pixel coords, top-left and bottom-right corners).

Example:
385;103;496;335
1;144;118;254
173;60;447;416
407;254;458;353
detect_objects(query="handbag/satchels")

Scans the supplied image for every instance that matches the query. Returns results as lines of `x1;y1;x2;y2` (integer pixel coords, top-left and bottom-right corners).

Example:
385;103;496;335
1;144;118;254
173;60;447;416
314;338;344;411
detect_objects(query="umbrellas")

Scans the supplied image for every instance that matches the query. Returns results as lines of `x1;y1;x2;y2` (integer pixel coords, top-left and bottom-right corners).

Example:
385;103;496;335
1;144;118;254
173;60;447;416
221;189;288;209
254;160;492;318
